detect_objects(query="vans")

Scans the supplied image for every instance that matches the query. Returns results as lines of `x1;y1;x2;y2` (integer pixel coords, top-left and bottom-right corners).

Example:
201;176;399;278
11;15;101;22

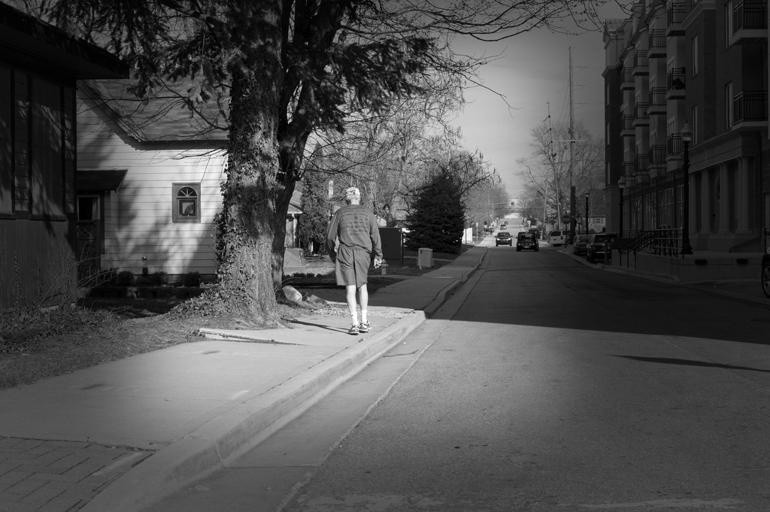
550;230;571;246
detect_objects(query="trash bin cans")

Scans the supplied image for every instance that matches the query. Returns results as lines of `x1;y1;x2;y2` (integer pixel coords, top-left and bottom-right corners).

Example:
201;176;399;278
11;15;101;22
417;247;433;268
574;241;587;256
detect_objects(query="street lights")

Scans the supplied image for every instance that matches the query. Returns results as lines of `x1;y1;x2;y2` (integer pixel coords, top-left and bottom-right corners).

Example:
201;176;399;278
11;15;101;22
618;175;626;236
584;190;590;234
680;122;693;255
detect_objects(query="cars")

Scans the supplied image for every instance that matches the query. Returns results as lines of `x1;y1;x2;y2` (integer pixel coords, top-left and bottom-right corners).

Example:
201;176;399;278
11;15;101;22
494;232;513;246
516;231;539;252
500;224;506;229
573;233;618;263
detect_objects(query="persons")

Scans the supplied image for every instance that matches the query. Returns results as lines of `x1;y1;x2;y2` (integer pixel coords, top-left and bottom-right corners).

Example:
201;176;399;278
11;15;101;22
326;187;383;334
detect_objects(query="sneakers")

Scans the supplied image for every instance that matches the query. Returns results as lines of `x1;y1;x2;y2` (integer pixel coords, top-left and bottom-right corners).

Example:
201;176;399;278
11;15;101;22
349;323;370;334
